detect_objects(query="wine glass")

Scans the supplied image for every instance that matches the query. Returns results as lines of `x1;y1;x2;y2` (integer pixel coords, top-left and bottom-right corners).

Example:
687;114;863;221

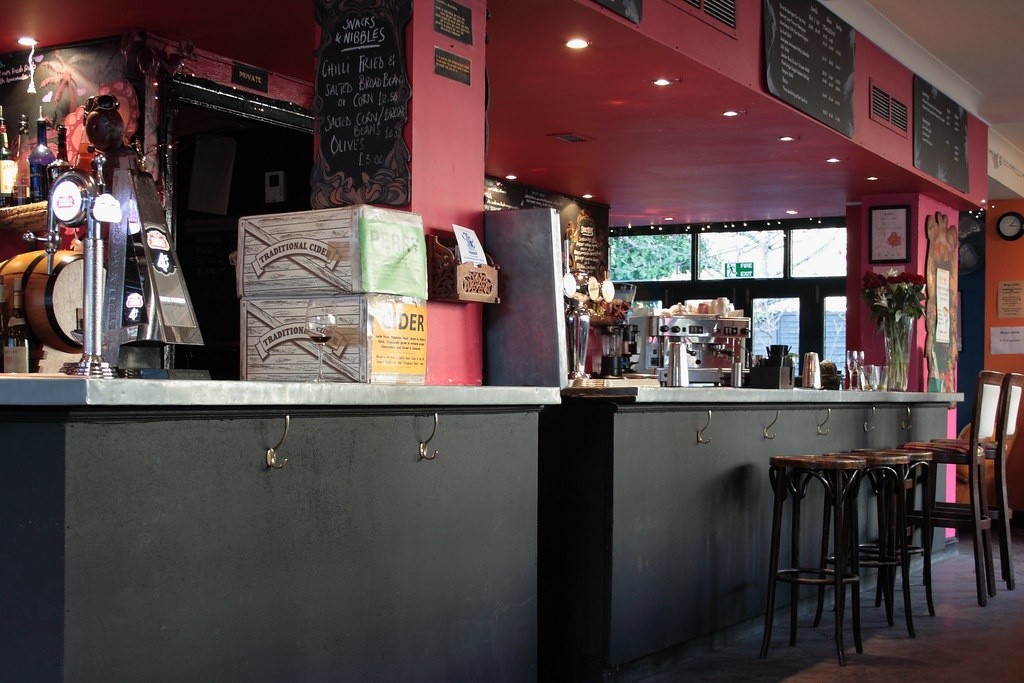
305;298;338;382
846;349;858;391
853;351;865;391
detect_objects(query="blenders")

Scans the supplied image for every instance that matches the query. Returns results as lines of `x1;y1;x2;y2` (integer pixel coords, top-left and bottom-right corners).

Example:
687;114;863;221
598;325;621;379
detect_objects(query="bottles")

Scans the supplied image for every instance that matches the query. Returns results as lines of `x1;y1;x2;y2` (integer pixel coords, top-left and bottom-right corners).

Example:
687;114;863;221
0;274;11;373
0;117;17;208
3;278;30;373
28;118;55;201
73;124;94;178
12;114;31;204
45;125;72;201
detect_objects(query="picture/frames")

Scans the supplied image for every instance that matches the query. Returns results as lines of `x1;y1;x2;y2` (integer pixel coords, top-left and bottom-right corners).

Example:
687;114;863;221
868;205;910;262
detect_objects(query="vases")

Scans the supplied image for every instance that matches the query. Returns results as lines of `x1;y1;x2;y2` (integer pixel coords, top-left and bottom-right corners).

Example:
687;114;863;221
883;313;914;392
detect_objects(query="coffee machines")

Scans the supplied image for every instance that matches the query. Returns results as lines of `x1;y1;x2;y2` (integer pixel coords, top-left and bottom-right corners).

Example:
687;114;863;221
622;324;642;374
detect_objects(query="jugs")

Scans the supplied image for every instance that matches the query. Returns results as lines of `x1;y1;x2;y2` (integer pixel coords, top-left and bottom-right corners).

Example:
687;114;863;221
667;342;689;387
779;354;796;389
802;351;821;390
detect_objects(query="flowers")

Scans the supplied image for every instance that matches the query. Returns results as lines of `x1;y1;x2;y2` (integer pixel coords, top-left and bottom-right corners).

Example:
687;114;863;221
860;267;926;335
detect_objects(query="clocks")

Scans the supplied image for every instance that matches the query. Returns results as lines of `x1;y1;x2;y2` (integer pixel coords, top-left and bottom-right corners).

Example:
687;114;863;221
995;211;1024;241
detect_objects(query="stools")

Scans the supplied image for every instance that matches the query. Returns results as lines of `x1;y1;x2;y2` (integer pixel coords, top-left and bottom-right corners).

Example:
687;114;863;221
757;453;865;668
813;452;916;638
851;448;936;618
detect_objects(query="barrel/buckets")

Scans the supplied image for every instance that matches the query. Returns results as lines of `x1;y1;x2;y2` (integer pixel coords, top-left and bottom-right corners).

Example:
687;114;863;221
1;250;107;355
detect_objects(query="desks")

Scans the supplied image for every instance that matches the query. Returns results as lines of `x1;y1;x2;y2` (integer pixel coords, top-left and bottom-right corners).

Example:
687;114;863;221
0;373;563;683
538;385;966;682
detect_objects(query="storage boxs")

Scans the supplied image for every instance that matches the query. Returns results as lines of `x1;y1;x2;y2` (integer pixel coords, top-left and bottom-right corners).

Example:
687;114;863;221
239;296;427;387
236;205;428;296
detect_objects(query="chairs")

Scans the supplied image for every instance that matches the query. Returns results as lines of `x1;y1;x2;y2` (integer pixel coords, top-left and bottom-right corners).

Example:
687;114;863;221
898;369;1005;608
922;368;1024;591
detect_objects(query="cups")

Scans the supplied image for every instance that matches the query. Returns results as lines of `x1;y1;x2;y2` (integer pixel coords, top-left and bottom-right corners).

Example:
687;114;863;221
698;297;729;315
859;365;889;392
731;362;742;387
